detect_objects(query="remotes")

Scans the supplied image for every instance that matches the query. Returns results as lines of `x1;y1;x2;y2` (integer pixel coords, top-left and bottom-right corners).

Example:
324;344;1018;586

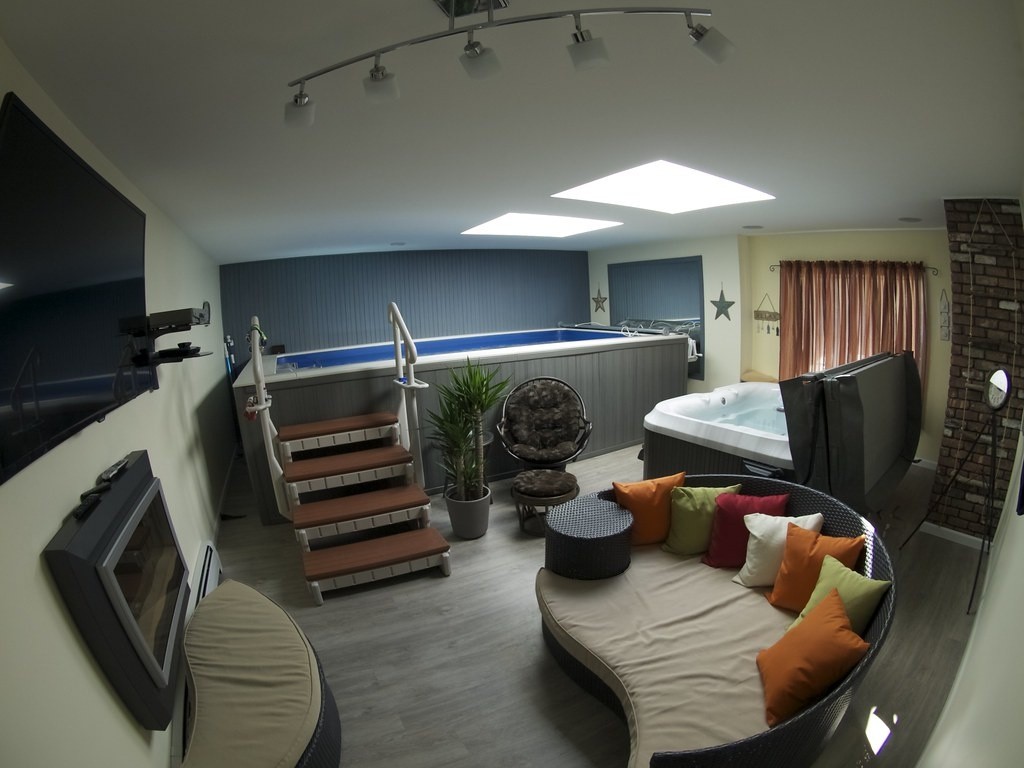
72;459;129;521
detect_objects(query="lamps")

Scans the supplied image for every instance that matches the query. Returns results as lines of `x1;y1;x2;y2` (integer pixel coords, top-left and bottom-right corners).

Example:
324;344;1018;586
289;10;711;106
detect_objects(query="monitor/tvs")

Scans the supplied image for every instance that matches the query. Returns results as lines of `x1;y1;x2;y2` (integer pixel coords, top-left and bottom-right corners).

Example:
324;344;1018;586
95;476;190;690
0;91;155;483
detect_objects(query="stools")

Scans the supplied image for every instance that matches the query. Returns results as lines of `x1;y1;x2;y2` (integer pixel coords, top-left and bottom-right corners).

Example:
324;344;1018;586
509;470;580;536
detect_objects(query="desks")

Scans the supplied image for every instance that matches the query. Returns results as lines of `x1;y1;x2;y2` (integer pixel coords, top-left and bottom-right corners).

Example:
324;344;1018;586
545;499;634;580
431;429;496;505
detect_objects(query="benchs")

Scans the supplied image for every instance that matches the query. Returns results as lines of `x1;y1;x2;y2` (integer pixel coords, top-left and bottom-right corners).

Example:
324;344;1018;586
178;579;343;768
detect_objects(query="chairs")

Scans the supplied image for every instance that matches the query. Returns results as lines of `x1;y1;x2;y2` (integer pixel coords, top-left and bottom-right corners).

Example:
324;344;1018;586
496;375;592;468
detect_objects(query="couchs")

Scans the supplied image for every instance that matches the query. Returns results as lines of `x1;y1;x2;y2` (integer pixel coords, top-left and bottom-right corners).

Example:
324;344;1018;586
535;474;896;768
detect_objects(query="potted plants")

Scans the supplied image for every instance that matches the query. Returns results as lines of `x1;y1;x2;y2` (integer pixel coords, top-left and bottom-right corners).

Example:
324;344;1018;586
429;358;514;542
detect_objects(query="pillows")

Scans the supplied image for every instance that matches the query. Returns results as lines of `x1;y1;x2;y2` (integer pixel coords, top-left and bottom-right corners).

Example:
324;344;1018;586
784;554;892;638
763;522;867;613
731;512;824;587
754;589;870;729
699;493;791;567
661;482;741;553
612;471;688;547
